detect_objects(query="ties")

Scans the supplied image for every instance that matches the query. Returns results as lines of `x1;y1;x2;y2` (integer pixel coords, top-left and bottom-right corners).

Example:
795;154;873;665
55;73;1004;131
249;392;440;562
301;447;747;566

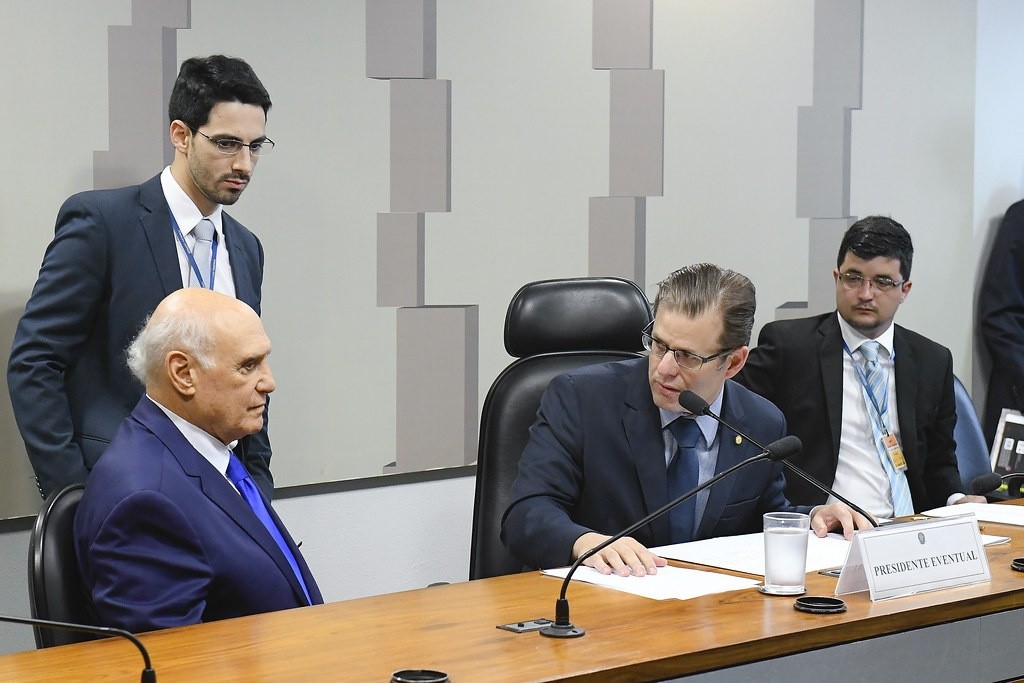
225;449;313;606
860;340;915;518
188;219;216;290
666;416;702;545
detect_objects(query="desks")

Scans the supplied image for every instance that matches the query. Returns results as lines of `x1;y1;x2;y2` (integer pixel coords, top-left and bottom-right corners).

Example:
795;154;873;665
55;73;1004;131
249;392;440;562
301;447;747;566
0;496;1023;682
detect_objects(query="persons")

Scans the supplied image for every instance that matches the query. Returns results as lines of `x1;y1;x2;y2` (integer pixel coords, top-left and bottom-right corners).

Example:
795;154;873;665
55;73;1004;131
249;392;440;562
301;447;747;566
730;217;991;521
73;287;326;635
8;57;277;506
978;199;1024;416
500;265;873;575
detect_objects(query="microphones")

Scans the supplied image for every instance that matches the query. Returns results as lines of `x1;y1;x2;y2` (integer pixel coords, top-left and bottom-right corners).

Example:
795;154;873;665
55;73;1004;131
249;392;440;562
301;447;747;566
971;471;1024;495
540;436;802;638
676;390;880;528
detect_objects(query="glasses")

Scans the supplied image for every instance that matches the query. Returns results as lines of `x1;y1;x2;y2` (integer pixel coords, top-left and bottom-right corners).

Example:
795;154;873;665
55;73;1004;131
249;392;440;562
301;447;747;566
839;269;905;293
642;318;736;371
184;122;275;156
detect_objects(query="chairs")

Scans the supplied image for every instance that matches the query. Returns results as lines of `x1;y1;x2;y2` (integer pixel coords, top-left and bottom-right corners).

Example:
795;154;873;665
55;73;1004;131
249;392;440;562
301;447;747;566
27;482;101;651
944;377;994;496
466;276;662;584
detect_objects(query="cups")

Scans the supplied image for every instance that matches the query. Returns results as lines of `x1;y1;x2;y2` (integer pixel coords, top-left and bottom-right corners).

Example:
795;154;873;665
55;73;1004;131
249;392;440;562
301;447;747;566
763;512;810;593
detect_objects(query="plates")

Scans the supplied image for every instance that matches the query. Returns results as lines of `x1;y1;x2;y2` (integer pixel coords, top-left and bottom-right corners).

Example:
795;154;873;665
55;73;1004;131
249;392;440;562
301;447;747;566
757;586;807;595
